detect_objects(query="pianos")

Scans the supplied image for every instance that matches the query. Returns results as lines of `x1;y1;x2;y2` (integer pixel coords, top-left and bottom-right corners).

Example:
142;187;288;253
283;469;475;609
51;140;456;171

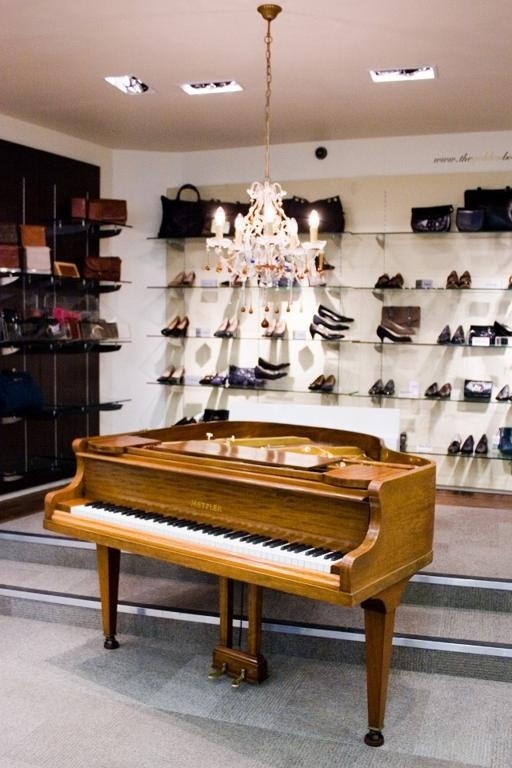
44;420;436;747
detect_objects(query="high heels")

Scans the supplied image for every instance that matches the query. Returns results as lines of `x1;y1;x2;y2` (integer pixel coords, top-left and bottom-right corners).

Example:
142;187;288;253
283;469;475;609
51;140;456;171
378;318;415;342
310;305;353;340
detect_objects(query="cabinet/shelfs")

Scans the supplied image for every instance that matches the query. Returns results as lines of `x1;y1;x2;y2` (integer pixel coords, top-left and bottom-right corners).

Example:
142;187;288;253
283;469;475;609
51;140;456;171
145;231;512;461
1;217;121;422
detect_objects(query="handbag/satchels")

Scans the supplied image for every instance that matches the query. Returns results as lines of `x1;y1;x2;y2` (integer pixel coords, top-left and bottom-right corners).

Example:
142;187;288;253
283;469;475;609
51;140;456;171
457;188;512;231
159;185;251;237
89;198;127;224
412;204;452;231
78;319;118;341
463;379;492;402
85;256;121;280
284;195;345;231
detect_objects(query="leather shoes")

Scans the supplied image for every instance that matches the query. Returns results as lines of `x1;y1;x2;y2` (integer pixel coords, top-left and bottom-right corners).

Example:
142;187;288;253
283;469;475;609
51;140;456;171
425;383;451;399
376;273;404;289
447;270;471;288
437;321;512;344
309;374;335;390
496;386;509;400
169;271;194;286
158;364;184;383
199;357;289;386
162;316;189;336
314;255;334;271
214;319;239;337
369;379;395;396
447;434;512;455
265;319;287;337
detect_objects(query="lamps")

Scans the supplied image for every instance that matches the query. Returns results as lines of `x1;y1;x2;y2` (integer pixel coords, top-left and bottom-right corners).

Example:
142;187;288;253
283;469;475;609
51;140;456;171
205;3;328;314
